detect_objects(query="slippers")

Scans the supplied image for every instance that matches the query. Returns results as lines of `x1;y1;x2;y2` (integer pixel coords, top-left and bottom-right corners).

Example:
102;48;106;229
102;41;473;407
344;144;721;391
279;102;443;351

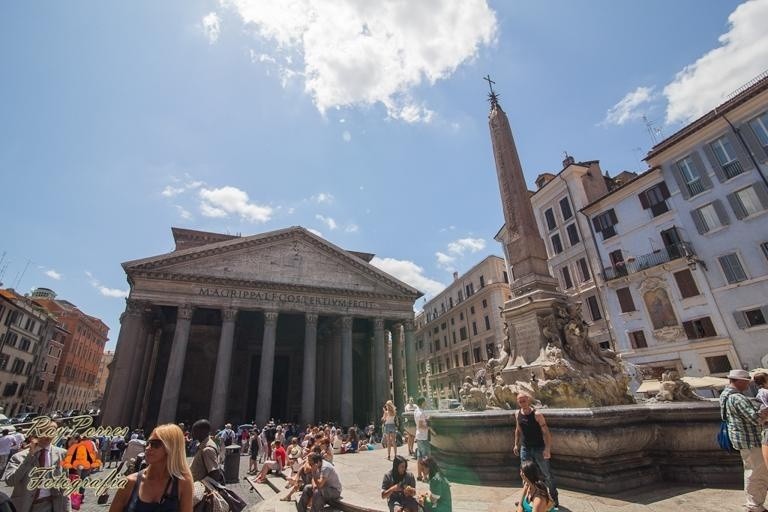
280;496;291;500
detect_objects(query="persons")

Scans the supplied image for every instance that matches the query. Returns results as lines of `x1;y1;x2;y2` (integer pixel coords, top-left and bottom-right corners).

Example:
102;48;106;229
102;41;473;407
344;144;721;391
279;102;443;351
513;395;559;507
515;463;557;512
720;370;768;512
0;397;452;511
755;372;768;473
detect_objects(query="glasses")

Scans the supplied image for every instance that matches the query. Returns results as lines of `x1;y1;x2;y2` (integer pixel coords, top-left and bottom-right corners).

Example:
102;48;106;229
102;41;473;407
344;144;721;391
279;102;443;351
146;440;167;450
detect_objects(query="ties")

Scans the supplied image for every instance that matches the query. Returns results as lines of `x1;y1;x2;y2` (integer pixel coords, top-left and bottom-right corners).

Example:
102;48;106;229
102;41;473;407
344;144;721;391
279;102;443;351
36;450;45;498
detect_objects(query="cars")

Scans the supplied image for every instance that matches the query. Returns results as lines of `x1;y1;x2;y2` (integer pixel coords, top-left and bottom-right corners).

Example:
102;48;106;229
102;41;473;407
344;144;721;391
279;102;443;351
0;407;101;428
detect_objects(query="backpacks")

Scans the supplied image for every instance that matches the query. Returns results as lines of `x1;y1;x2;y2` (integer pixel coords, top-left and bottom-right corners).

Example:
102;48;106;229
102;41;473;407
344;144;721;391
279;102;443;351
224;430;232;446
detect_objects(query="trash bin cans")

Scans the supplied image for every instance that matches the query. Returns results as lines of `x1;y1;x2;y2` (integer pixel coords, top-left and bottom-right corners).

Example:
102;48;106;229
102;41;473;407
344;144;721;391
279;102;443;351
223;444;242;484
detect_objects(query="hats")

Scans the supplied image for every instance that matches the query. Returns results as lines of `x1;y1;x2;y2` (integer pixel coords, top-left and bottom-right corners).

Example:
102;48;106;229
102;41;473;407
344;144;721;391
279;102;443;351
225;424;231;428
726;370;752;381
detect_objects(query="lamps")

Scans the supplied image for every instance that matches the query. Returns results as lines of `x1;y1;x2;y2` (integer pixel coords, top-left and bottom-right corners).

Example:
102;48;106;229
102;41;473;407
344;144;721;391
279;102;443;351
687;258;707;271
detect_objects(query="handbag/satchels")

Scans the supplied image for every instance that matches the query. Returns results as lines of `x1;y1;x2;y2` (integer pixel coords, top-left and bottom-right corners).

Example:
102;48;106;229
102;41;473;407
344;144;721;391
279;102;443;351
717;421;733;449
193;477;246;512
